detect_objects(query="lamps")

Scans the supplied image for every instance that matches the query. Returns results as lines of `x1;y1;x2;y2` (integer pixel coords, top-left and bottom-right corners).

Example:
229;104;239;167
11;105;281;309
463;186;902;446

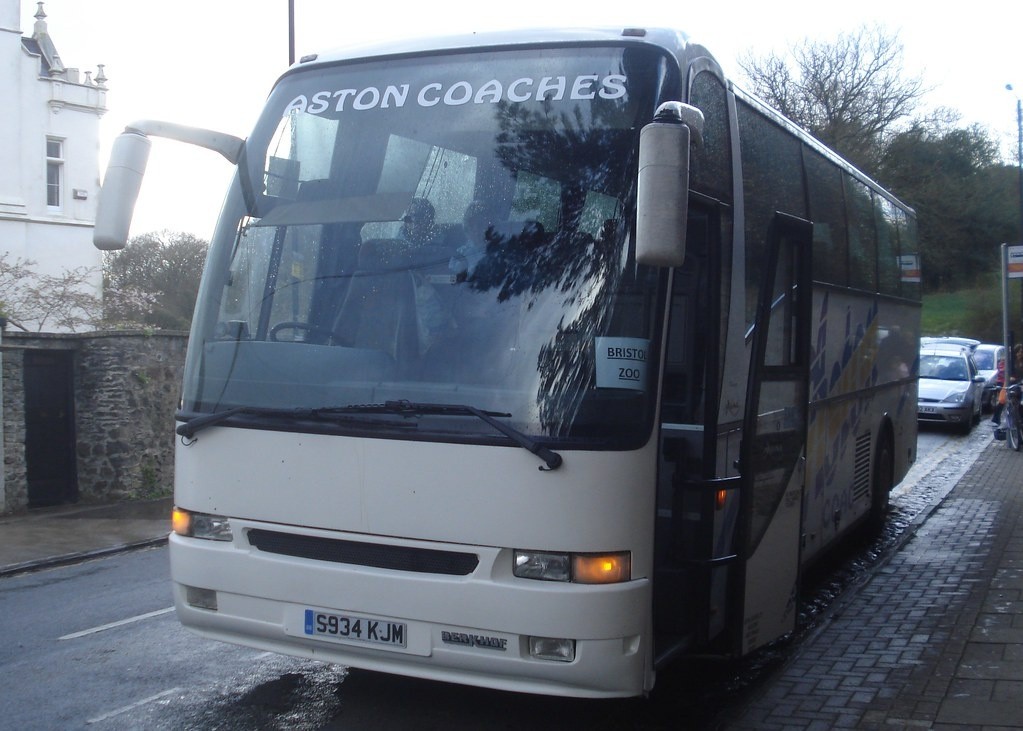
73;189;87;200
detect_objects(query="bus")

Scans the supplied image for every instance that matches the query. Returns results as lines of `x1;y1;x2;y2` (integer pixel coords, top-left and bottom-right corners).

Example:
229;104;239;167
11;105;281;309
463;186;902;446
96;28;919;699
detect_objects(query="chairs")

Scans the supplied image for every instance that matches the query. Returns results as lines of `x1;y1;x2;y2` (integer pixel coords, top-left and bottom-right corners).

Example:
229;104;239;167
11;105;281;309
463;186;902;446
325;220;592;379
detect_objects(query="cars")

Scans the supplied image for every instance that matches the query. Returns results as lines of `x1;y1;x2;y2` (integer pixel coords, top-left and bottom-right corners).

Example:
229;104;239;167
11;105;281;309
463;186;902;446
972;344;1005;409
918;337;986;435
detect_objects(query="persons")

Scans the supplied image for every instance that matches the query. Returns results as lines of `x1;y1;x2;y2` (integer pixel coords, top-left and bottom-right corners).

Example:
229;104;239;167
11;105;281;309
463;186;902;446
992;344;1023;435
403;198;503;329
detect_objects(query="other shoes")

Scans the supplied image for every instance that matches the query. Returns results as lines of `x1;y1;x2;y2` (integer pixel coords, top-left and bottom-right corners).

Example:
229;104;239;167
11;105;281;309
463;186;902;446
985;422;999;427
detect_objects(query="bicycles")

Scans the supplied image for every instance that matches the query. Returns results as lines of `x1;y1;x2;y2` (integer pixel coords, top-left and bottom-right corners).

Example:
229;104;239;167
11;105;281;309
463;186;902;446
988;381;1023;452
